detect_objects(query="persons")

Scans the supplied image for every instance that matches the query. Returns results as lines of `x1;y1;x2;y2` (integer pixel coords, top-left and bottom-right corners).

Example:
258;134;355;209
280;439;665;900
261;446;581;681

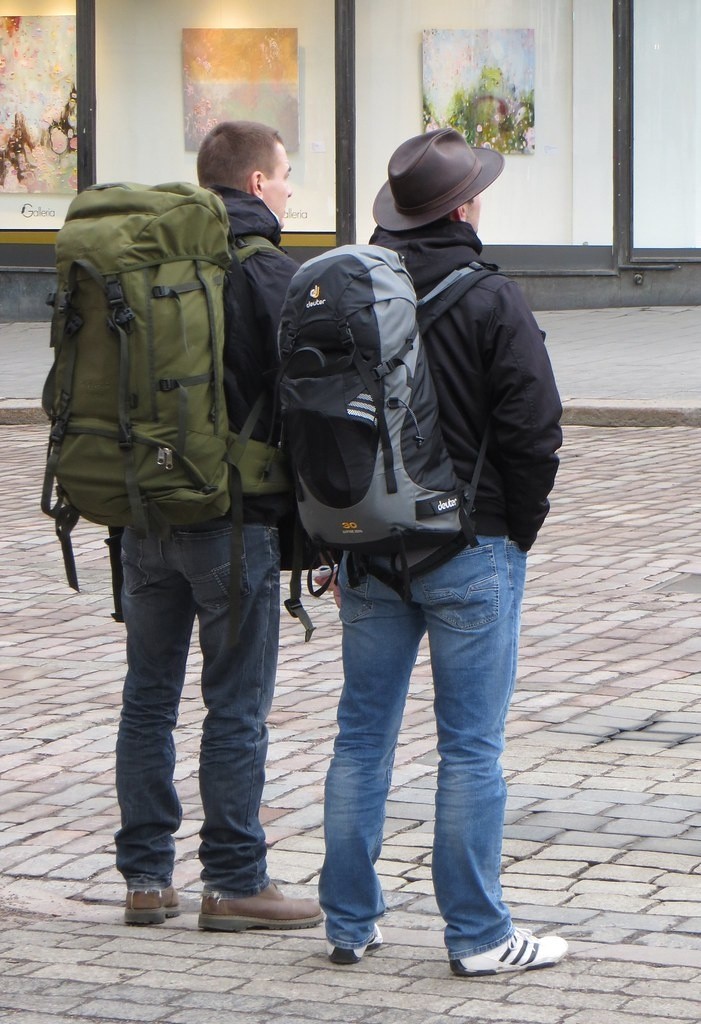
113;121;322;933
315;128;570;973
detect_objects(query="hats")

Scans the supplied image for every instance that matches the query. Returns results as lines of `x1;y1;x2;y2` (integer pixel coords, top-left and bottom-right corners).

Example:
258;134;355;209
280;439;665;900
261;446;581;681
374;129;504;231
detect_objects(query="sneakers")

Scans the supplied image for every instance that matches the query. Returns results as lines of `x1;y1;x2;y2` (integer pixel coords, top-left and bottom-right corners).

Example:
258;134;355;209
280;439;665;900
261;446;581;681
448;925;569;977
325;919;384;965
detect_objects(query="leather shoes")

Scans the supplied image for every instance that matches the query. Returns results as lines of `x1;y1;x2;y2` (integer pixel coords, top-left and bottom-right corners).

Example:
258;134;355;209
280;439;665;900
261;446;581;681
197;881;325;932
124;885;182;923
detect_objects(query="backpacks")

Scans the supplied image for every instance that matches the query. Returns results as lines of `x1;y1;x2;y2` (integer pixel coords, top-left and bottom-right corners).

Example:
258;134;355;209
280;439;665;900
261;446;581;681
276;243;502;642
40;183;286;647
